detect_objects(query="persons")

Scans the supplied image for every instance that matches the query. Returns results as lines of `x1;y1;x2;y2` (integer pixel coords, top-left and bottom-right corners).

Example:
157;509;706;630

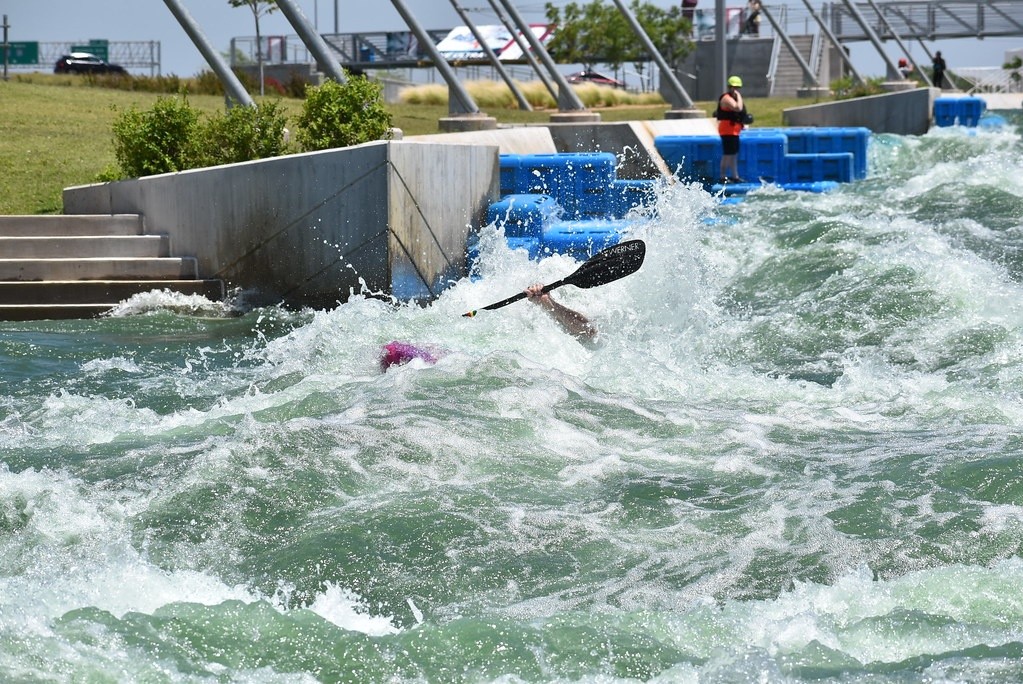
525;285;596;342
719;76;746;184
747;0;762;37
898;58;913;78
930;51;946;88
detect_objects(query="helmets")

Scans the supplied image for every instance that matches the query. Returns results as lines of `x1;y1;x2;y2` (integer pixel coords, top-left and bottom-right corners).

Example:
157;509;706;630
899;59;907;66
728;76;743;87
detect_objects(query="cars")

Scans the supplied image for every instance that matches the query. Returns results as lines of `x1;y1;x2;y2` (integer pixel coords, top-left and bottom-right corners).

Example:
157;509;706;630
564;69;625;90
53;52;125;76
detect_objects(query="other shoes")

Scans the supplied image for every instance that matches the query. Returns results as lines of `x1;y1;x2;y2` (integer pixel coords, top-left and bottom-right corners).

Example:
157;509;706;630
731;177;745;183
716;179;731;183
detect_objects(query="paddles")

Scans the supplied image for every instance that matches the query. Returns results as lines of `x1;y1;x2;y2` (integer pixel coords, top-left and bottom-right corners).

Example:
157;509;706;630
461;240;647;318
